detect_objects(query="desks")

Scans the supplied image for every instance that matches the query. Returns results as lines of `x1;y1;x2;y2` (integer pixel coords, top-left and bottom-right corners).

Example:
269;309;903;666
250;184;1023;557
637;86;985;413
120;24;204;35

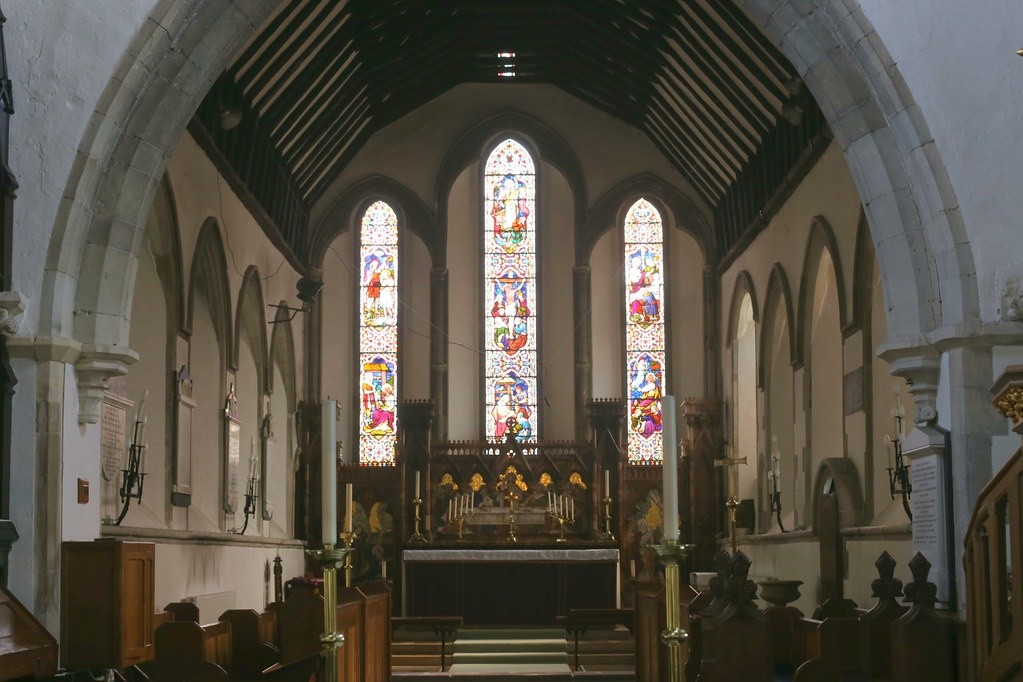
570;608;636;671
392;616;462;672
401;533;621;630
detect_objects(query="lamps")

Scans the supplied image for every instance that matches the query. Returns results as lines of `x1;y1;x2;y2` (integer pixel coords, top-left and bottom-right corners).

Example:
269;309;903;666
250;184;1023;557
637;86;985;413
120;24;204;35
120;388;149;504
768;434;782;516
781;101;804;127
216;100;243;130
267;276;325;324
883;380;912;501
244;438;260;520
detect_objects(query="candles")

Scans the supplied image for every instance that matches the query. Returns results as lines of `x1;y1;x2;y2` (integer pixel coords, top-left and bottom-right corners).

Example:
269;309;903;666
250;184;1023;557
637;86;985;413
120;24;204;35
448;491;475;521
382;561;386;579
548;492;574;520
662;393;679;540
346;482;352;533
605;469;609;497
322;396;338;545
416;471;420;499
630;559;635;577
